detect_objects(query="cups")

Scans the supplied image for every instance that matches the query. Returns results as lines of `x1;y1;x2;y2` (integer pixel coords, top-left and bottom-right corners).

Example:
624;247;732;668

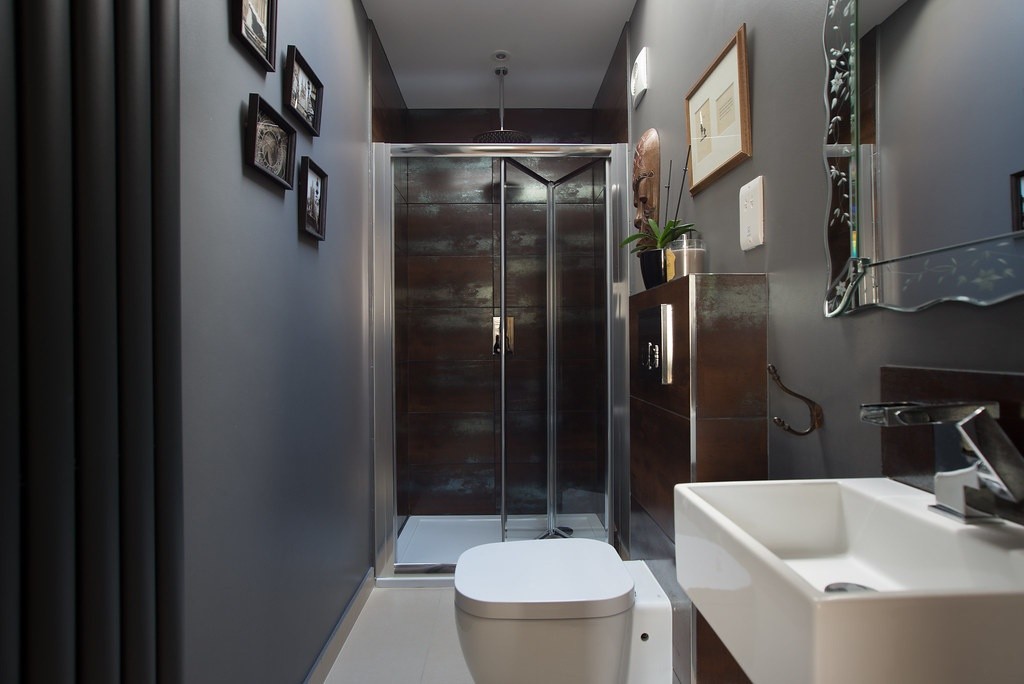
668;239;705;281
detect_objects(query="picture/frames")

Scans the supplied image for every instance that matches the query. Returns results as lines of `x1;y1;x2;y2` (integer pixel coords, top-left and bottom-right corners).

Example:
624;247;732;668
299;156;328;242
283;44;324;137
685;23;754;198
227;0;278;73
245;92;297;191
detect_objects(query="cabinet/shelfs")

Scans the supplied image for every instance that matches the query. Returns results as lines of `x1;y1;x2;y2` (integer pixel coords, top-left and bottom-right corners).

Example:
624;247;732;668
627;272;769;559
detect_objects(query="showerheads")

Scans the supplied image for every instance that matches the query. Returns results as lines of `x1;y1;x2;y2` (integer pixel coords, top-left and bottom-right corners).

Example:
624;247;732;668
473;129;532;143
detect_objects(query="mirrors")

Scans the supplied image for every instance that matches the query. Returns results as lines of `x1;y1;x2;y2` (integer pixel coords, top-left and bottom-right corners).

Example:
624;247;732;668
821;0;1024;319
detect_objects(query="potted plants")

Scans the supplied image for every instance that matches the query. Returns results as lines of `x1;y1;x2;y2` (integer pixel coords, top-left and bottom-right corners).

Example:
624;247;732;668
619;218;697;290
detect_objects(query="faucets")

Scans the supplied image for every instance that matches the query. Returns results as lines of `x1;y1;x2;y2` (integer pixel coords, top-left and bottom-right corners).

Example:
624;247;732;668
927;406;1024;525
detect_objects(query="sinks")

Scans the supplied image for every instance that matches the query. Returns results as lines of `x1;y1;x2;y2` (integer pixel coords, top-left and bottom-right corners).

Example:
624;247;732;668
673;476;1024;684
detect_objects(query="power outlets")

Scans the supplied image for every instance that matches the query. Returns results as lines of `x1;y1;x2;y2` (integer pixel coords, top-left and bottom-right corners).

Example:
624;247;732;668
739;176;764;251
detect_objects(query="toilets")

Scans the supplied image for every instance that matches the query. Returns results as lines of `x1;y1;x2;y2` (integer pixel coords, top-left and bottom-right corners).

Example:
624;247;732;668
452;537;638;684
